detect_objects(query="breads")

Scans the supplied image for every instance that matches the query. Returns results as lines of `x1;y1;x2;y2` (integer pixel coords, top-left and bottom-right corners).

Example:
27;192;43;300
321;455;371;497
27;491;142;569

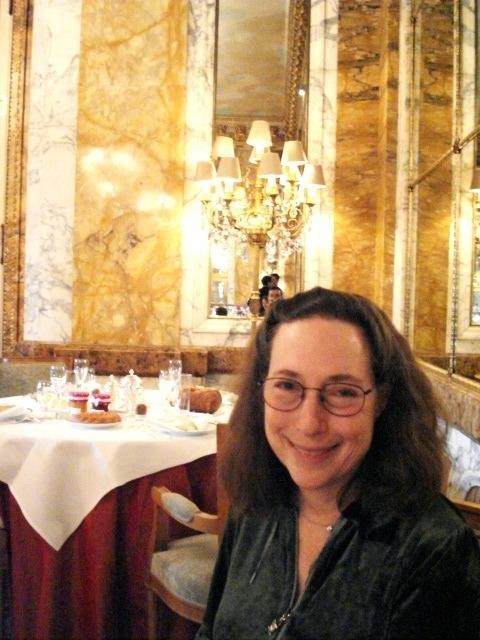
73;410;122;425
178;387;221;415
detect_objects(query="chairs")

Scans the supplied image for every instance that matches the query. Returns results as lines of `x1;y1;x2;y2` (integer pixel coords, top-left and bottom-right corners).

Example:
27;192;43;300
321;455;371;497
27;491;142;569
146;425;231;638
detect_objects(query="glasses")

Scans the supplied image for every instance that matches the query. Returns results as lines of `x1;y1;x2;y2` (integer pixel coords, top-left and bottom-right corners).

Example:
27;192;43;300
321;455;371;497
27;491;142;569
255;368;379;419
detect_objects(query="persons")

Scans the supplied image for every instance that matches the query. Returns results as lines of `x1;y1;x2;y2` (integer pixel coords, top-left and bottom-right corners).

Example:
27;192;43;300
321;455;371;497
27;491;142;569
259;275;273;313
271;273;281;288
257;294;269;318
267;286;284;305
186;280;480;638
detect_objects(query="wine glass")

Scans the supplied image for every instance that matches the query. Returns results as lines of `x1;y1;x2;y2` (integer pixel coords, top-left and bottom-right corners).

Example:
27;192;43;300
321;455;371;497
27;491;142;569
35;358;192;423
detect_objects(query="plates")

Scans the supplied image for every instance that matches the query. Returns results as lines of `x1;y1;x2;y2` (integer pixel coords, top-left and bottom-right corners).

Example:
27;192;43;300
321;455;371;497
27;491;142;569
158;421;216;437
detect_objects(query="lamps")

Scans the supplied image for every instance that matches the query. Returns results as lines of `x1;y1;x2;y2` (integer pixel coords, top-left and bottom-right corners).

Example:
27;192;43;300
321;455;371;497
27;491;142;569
193;117;326;337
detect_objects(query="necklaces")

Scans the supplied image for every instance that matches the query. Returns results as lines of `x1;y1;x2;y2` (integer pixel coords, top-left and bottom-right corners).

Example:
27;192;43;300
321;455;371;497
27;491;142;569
297;507;338;533
